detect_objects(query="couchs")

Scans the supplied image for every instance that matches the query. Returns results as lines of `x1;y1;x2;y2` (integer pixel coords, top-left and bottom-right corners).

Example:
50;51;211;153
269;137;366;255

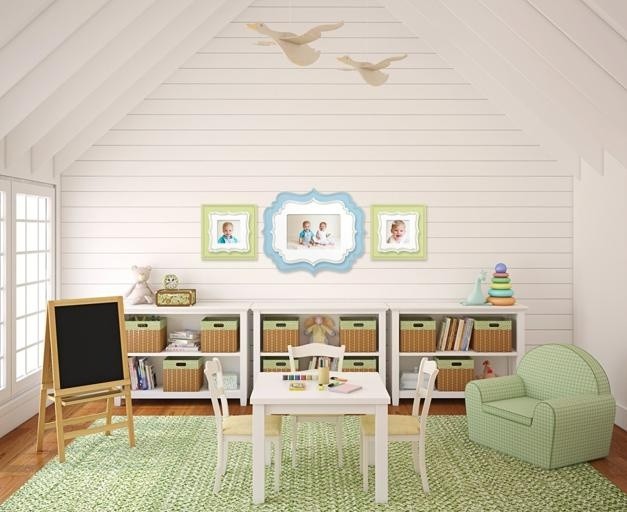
464;342;616;471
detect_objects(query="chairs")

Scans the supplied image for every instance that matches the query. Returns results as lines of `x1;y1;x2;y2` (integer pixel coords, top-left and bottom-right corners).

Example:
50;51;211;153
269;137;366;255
287;344;346;467
359;357;440;493
202;357;282;493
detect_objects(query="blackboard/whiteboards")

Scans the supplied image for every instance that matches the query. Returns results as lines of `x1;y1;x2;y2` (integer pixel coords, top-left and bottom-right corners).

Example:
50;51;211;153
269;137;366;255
35;296;135;464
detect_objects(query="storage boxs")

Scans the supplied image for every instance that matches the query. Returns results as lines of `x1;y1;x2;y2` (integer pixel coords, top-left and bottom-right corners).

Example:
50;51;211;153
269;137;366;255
157;289;196;305
342;358;377;372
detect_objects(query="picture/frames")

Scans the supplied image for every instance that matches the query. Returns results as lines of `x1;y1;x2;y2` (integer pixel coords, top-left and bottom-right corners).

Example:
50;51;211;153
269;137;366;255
261;187;366;277
201;204;259;262
371;204;428;262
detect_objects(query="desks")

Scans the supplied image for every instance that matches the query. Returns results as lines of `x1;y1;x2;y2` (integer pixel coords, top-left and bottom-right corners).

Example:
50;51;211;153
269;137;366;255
250;372;391;504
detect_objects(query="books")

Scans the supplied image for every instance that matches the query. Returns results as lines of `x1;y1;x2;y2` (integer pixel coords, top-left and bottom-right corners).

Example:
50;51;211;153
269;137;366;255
307;355;338;371
399;357;475;391
128;356;157;390
165;329;199;352
328;383;362;394
436;315;475;352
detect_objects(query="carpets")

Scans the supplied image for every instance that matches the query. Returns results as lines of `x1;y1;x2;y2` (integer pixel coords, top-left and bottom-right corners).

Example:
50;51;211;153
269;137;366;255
0;415;627;511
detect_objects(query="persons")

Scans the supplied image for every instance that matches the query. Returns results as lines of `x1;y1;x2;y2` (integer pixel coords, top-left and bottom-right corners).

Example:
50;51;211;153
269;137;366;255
219;222;238;244
298;221;314;247
314;222;335;245
387;220;410;244
303;315;337;346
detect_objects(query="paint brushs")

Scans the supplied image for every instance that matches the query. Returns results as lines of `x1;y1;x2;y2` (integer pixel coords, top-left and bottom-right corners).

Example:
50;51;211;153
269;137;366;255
329;376;347;381
328;381;342;386
319;355;329;367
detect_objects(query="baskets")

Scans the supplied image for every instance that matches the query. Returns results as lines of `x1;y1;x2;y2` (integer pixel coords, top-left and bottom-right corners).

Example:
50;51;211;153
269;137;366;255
342;361;377;373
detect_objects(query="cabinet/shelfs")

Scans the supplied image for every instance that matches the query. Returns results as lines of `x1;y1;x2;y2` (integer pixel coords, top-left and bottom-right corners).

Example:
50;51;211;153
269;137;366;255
112;302;254;407
387;302;529;407
249;302;389;389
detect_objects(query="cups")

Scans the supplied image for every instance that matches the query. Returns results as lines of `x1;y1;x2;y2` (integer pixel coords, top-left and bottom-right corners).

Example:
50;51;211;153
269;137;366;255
318;367;330;385
